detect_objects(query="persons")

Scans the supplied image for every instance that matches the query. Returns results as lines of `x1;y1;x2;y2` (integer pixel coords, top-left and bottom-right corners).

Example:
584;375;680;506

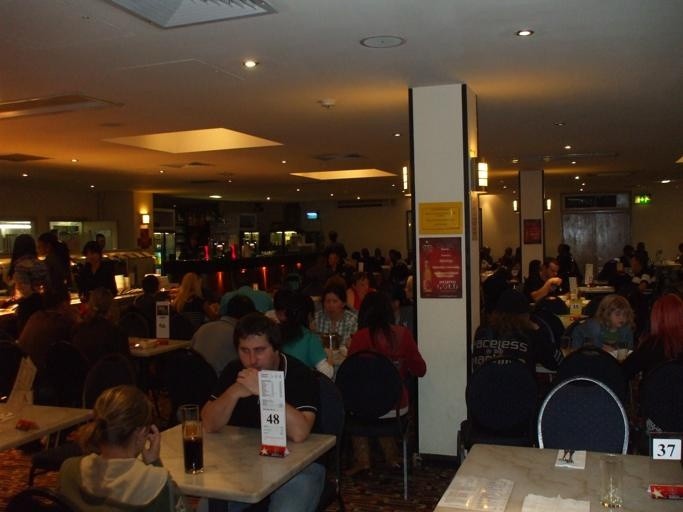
1;229;681;512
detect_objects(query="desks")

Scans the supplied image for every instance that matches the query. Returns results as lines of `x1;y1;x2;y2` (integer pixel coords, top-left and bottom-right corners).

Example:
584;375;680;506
0;403;95;451
134;421;336;512
535;349;639;387
126;336;191;418
434;444;683;511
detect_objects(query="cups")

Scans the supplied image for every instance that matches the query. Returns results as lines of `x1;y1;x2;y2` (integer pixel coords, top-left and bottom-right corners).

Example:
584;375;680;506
180;404;199;428
182;423;204;475
599;455;624;509
559;333;629;363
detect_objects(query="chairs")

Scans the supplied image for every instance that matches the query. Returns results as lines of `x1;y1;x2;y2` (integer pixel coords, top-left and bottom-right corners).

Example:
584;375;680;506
335;351;411;500
5;488;80;512
119;305;164;418
558;347;628;406
536;378;629;455
638;360;683;451
46;341;91;447
29;353;135;485
311;372;347;512
456;357;538;471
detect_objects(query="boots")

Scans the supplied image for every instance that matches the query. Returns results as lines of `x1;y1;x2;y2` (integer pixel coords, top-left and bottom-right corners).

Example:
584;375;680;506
342;427;371;478
377;435;410;482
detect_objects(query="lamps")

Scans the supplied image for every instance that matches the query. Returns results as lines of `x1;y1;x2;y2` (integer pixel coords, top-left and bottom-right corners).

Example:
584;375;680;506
402;166;408;189
124;277;130;289
546;198;552;210
114;274;124;290
477;157;490;191
143;214;150;223
512;200;518;213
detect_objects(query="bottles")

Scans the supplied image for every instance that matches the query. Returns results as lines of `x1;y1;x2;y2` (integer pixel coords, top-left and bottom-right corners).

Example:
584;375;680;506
75;263;81;282
